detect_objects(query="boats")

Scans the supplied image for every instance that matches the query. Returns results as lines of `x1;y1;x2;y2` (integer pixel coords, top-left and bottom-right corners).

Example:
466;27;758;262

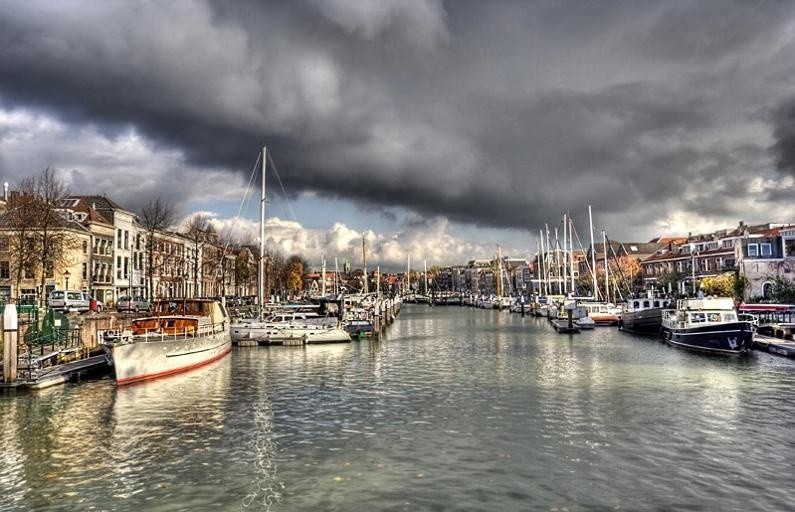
737;302;795;324
22;353;108;390
752;323;795;357
660;252;760;357
104;312;232;387
618;277;673;336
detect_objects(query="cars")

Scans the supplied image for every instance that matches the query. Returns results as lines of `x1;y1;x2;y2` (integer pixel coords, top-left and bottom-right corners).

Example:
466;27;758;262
115;295;152;313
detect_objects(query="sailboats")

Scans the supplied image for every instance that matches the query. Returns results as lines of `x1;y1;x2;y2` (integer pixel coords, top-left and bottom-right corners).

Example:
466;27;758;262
206;145;354;347
340;205;623;339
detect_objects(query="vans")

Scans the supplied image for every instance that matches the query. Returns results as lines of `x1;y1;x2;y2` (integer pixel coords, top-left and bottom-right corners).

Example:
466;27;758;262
47;289;104;314
